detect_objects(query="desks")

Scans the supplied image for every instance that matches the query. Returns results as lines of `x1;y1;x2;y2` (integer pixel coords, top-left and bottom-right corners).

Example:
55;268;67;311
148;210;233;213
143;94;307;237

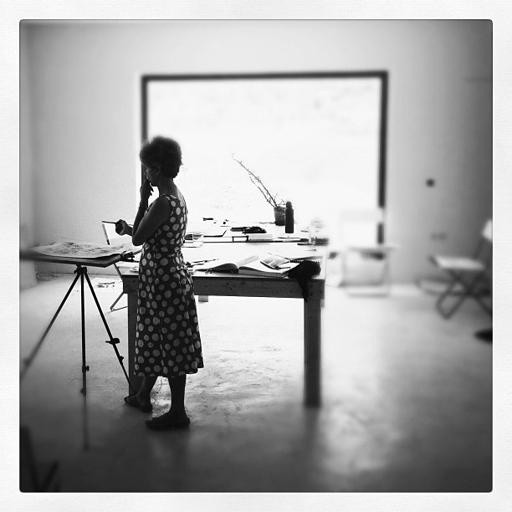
20;241;136;404
120;223;329;408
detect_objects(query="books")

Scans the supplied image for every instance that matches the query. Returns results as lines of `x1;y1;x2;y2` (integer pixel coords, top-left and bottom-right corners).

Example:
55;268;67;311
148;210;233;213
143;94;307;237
196;254;300;279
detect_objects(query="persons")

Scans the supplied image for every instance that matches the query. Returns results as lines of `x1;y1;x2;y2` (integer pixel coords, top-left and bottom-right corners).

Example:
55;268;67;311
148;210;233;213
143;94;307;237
115;137;204;431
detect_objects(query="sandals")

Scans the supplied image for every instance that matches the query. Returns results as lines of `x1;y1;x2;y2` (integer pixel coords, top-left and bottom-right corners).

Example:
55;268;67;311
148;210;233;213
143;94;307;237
124;396;153;412
145;412;190;429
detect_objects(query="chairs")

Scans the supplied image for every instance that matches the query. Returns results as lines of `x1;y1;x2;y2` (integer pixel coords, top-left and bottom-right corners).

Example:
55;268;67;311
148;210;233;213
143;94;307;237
429;218;493;319
100;219;143;313
337;205;398;297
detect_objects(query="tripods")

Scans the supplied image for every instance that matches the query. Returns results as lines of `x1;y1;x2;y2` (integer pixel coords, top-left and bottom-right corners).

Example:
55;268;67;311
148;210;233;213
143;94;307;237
20;264;132;393
